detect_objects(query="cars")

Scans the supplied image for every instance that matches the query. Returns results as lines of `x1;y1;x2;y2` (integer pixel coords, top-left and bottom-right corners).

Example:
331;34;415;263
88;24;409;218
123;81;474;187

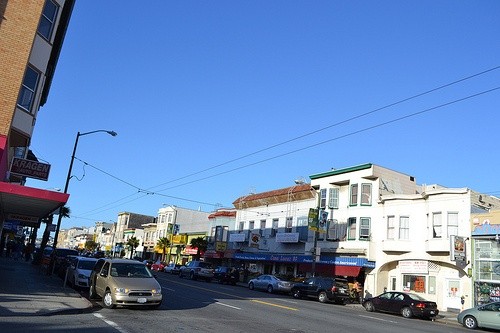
362;291;439;318
457;299;500;330
247;274;296;294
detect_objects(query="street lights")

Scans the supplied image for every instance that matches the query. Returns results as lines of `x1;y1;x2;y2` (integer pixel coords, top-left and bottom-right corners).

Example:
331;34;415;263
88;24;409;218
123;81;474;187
47;187;62;191
51;129;118;251
294;179;321;275
162;203;177;263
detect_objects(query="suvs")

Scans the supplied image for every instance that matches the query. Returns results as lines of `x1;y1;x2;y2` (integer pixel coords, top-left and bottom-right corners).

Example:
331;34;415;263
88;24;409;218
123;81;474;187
31;244;163;310
92;251;105;259
131;256;215;282
214;266;239;286
290;277;350;306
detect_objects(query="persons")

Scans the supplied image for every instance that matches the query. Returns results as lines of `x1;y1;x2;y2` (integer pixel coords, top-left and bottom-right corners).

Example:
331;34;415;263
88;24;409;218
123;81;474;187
243;266;250;283
25;242;33;262
352;279;362;303
230;265;237;286
146;256;153;271
5;240;13;256
